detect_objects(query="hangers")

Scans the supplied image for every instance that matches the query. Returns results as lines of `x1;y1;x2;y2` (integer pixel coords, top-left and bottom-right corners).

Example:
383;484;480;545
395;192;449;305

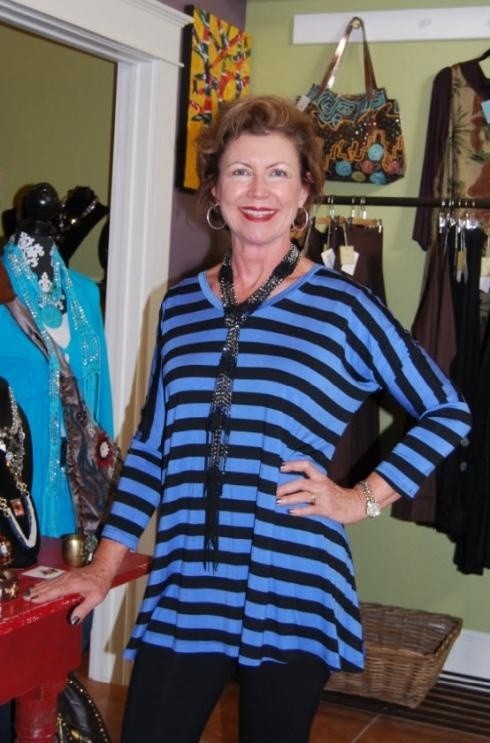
478;48;490;67
435;198;477;246
311;195;383;233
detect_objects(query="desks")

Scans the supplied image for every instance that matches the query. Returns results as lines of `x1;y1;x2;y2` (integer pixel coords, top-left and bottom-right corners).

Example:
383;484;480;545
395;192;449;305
0;534;153;743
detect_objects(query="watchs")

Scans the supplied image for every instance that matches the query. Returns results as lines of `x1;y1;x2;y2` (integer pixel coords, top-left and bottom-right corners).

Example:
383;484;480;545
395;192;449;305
354;478;381;520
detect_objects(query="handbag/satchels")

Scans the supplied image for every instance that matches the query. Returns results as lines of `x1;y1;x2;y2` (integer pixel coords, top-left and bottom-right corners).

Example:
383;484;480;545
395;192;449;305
296;83;406;185
61;378;122;531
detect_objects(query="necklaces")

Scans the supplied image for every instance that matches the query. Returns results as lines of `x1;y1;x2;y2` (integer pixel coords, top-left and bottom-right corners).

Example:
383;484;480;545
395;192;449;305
0;384;37;549
56;195;99;241
7;231;99;482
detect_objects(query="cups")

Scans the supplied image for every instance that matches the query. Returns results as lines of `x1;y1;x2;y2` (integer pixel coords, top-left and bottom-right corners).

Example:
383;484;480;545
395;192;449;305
60;532;89;568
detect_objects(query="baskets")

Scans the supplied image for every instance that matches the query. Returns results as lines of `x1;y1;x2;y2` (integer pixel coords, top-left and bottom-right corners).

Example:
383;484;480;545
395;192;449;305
323;602;463;710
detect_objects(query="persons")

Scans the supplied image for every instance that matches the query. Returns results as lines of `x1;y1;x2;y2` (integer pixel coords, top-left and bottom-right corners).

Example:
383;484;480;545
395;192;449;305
51;185;110;327
1;377;42;570
2;184;116;538
20;94;479;743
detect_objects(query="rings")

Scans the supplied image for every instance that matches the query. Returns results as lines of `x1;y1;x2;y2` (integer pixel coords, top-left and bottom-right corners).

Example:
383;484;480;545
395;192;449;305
309;490;317;504
